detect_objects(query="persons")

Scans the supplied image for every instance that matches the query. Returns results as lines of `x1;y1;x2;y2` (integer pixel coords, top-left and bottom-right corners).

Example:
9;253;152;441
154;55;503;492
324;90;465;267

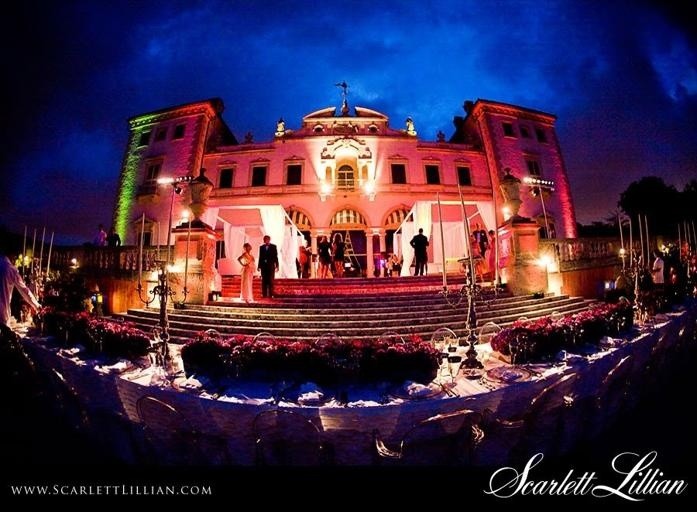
106;227;121;247
470;223;497;281
257;235;279;298
385;255;403;278
237;244;255;301
649;249;665;286
298;233;346;280
410;229;429;276
0;251;44;328
90;224;109;247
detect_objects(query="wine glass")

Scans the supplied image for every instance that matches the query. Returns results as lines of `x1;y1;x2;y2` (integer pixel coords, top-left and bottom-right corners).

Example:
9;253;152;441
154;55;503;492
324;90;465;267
433;336;460;386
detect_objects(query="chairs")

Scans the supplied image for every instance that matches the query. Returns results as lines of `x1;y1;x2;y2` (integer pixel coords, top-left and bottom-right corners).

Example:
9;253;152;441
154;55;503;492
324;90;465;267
0;301;697;512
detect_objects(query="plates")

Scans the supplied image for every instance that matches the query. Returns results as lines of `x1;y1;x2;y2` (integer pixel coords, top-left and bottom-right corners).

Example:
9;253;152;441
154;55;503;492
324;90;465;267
392;381;442;399
485;367;532;384
